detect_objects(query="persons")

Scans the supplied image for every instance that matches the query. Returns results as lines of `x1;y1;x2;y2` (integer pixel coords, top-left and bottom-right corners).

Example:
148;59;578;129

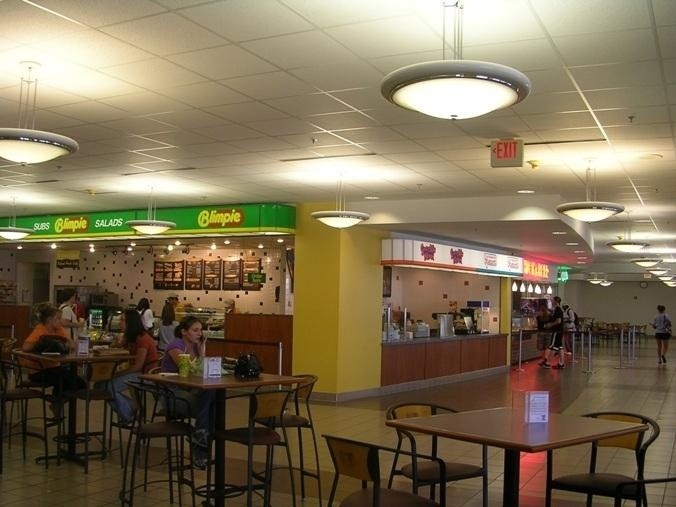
151;315;209;466
653;305;672;364
73;293;86;322
166;292;183;308
95;309;159;426
220;299;240;330
59;287;85;342
532;296;576;369
135;298;154;335
159;303;180;354
23;303;85;416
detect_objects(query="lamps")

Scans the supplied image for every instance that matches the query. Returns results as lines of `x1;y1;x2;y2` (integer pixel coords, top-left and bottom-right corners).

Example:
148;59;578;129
0;192;34;242
511;281;553;294
0;59;79;164
311;180;370;230
379;0;532;121
587;224;676;287
125;188;176;234
554;166;626;222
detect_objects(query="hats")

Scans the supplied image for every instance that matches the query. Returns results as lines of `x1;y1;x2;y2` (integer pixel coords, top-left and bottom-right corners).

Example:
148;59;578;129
167;293;178;299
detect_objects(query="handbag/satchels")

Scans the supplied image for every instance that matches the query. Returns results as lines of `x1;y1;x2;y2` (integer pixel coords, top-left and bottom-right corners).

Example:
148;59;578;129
567;309;579;325
235;355;261;377
663;315;672;334
33;334;69;356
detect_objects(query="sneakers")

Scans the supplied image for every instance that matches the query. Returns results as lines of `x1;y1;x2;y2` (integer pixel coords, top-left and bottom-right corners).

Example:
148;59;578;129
191;457;208;471
186;431;209;448
657;355;666;364
541;362;552;369
117;415;134;427
553;364;565;369
564;352;573;356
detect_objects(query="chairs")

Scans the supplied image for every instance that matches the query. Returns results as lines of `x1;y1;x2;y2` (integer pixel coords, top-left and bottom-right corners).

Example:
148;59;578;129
544;411;661;505
320;433;446;507
148;366;225;496
0;323;164;472
120;379;199;507
205;389;298;505
251;375;322;506
385;403;490;506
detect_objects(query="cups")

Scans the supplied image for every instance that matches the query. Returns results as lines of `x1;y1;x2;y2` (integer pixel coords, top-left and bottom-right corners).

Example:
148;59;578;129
177;353;190;378
